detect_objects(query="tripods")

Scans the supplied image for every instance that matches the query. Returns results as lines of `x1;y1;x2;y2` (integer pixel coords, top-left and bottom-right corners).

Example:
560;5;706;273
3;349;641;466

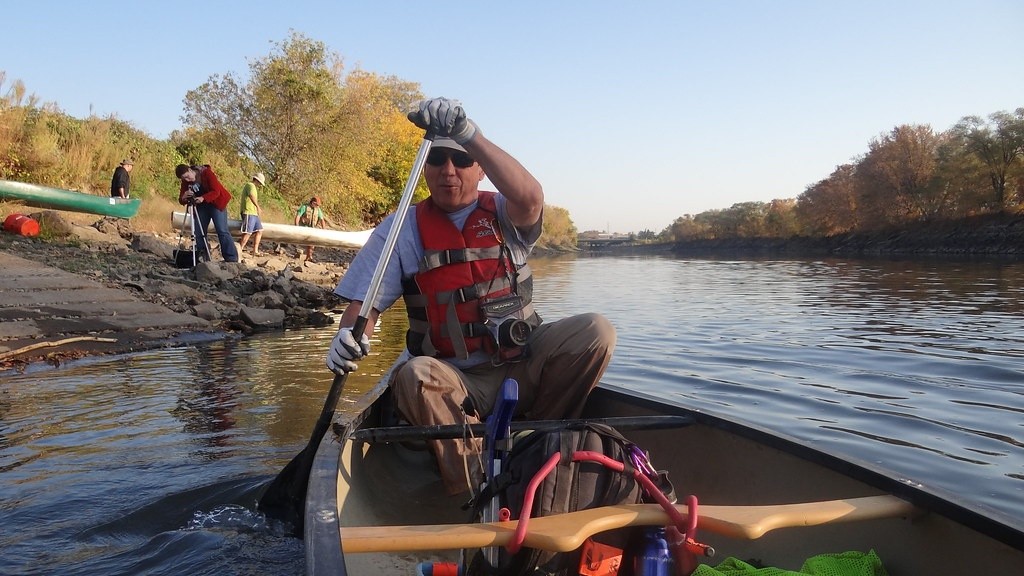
175;195;212;267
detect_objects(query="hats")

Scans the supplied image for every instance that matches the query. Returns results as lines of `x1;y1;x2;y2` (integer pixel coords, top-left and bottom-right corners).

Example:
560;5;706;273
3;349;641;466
430;118;483;153
253;173;265;186
120;159;135;166
311;196;320;205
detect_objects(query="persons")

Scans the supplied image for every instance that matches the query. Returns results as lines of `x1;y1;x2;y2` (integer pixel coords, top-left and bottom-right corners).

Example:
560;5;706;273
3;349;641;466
295;197;324;261
111;159;134;200
325;97;615;525
240;173;266;257
176;164;239;262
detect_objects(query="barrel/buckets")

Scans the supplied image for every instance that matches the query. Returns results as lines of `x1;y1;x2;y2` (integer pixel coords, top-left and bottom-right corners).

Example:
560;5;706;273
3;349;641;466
3;214;39;237
173;249;199;268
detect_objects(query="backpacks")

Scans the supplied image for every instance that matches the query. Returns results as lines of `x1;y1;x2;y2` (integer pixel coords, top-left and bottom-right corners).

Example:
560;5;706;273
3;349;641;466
471;420;678;576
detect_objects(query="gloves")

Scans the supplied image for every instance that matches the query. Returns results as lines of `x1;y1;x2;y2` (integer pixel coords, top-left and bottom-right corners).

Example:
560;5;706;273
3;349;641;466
407;98;477;143
326;327;370;375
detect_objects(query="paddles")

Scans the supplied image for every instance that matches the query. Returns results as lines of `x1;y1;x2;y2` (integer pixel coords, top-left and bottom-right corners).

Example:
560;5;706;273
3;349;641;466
261;110;454;514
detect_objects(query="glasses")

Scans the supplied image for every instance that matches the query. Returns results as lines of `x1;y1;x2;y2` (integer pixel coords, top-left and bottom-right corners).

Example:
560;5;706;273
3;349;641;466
425;151;475;169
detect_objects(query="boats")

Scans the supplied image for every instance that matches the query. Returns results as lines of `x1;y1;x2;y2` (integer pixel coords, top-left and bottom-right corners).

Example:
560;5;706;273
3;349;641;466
0;172;143;219
170;210;375;250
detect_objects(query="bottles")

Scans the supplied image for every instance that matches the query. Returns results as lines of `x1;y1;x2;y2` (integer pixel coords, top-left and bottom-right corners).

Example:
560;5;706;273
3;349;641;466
631;526;670;576
